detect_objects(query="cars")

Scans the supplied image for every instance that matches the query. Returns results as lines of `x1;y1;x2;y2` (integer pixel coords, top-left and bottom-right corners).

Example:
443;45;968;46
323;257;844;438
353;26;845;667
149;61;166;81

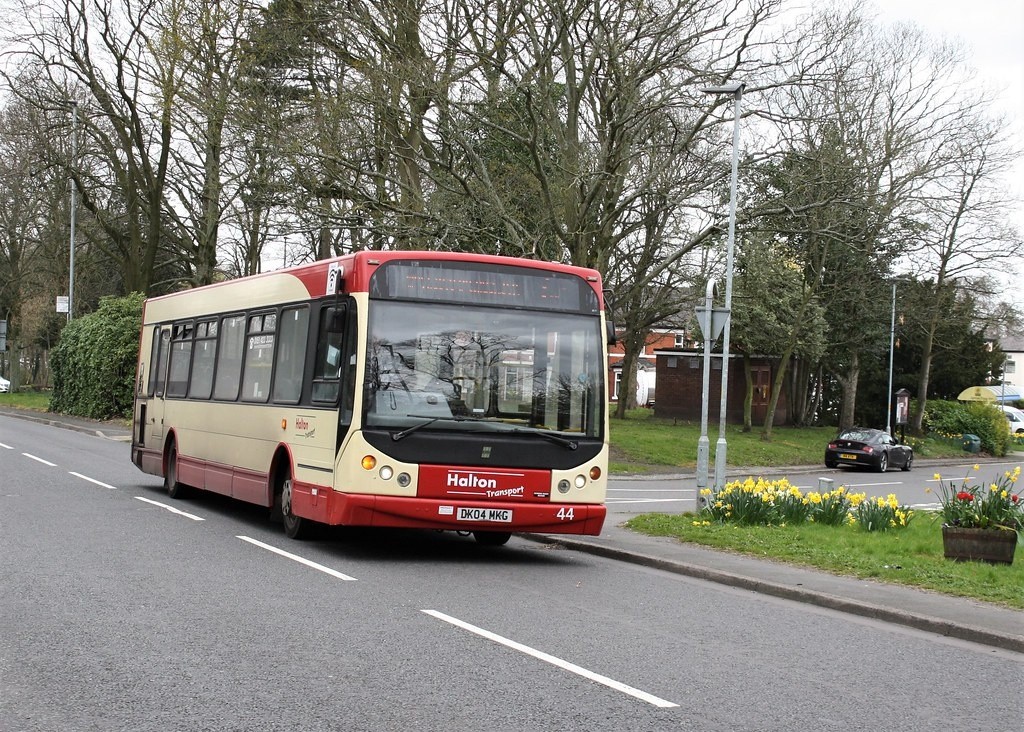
825;428;914;473
0;374;11;393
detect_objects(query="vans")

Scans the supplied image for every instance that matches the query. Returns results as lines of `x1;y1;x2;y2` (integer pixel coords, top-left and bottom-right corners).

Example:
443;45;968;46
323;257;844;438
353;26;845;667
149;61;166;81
992;404;1024;434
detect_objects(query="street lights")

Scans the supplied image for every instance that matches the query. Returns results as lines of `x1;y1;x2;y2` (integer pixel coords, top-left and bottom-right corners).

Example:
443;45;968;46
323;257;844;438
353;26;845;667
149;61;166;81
697;82;746;498
56;98;78;326
886;276;912;437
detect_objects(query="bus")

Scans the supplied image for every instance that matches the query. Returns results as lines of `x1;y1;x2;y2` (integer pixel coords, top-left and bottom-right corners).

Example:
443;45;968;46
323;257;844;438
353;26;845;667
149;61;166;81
129;250;618;545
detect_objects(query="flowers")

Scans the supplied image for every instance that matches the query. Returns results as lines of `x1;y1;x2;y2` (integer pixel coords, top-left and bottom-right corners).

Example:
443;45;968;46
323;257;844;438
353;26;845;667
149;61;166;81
927;464;1024;535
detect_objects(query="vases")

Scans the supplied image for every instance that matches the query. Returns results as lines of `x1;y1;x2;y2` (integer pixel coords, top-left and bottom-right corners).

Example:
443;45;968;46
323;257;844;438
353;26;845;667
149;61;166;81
942;524;1017;564
